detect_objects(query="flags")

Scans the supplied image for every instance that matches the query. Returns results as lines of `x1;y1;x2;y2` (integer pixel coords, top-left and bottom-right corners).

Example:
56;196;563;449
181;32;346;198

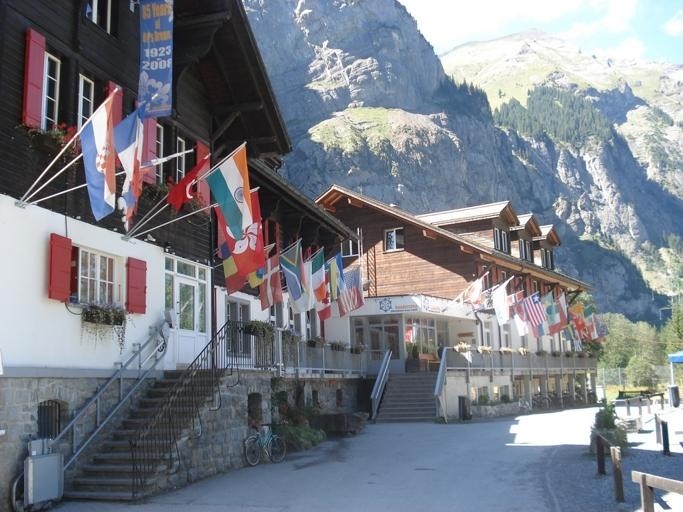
280;242;362;323
167;158;206;211
465;278;600;345
205;144;253;242
214;190;284;311
79;92;151;221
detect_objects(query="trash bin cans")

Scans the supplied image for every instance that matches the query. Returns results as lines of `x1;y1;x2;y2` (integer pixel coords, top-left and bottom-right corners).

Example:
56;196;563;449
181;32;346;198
670;387;679;407
458;397;471;421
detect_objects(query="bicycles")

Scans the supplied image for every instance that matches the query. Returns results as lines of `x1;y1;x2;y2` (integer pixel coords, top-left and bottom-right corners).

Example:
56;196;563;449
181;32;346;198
244;423;286;467
532;388;597;410
